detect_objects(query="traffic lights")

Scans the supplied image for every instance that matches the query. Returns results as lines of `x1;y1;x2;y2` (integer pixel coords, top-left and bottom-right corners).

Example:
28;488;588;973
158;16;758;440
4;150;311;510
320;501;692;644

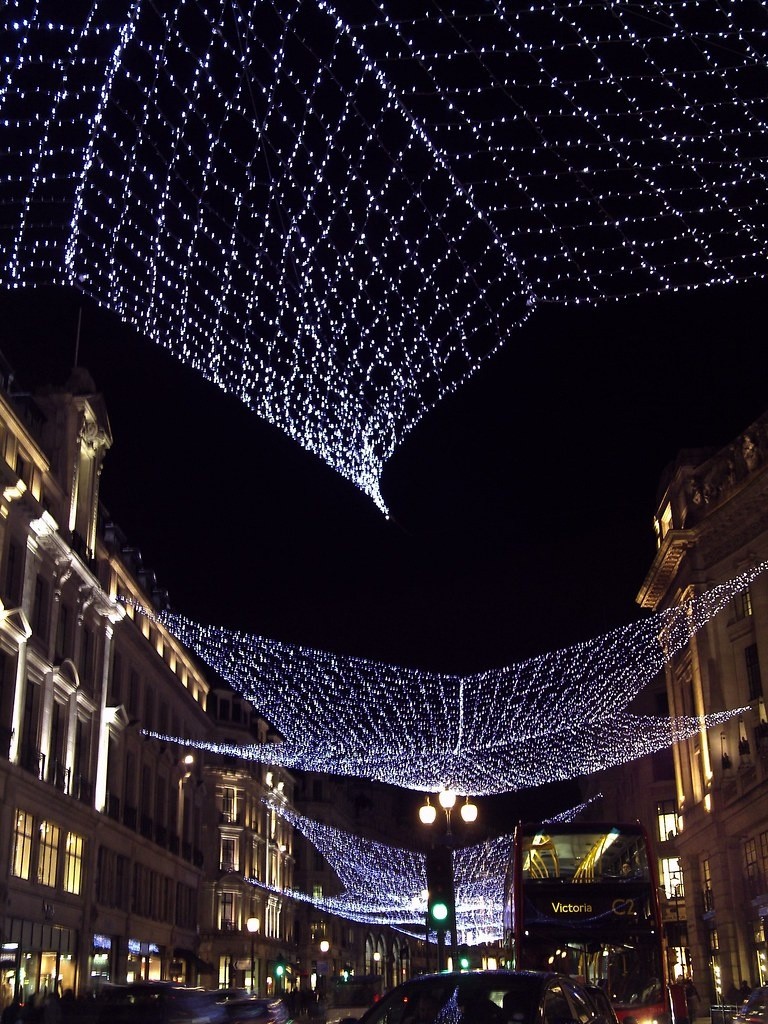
452;952;470;970
428;897;453;927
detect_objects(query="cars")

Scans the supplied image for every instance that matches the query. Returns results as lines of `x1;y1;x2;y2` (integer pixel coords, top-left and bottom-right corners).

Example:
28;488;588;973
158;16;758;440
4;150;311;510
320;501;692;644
732;987;768;1024
79;980;290;1024
325;971;619;1024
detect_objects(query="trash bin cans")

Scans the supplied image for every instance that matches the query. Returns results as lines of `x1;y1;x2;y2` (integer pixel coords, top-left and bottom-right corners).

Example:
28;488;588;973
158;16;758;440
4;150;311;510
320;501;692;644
665;980;691;1024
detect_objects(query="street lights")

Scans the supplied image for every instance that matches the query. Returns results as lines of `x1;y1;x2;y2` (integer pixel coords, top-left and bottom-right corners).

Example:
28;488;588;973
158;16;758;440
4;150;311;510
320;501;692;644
373;951;381;974
319;939;329;999
247;917;260;998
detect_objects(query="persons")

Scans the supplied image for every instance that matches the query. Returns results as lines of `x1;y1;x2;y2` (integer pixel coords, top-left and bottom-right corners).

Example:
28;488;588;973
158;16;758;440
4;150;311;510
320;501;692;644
620;863;634;876
267;978;320;1017
0;988;96;1024
676;967;751;1023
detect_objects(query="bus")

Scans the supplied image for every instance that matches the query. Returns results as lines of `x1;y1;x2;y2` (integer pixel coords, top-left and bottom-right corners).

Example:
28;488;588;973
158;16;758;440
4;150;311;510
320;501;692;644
502;820;683;1024
